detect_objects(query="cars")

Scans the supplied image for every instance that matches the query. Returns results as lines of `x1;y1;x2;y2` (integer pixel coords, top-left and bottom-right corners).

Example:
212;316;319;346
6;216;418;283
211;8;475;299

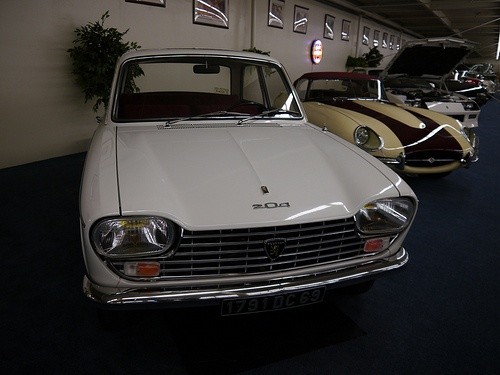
76;48;418;306
350;35;480;130
446;62;497;110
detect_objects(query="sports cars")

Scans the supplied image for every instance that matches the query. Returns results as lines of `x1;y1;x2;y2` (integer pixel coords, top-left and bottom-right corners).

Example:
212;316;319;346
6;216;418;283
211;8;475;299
272;71;475;175
